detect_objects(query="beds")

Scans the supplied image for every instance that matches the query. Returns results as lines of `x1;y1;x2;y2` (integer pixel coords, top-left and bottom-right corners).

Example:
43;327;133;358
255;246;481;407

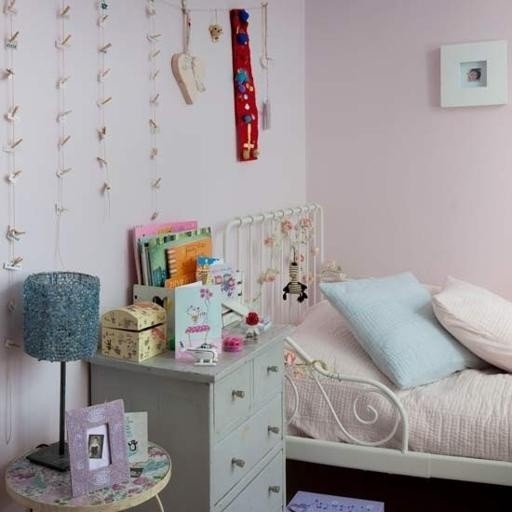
220;205;512;491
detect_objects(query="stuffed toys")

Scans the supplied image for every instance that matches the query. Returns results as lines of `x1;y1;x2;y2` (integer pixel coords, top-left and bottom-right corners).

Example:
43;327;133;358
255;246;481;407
282;260;308;302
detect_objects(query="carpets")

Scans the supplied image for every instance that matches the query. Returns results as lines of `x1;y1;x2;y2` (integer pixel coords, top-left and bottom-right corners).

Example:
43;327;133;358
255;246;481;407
284;491;384;512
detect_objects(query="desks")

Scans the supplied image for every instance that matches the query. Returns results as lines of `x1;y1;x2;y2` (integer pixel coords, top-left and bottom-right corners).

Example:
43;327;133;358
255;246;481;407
5;438;172;512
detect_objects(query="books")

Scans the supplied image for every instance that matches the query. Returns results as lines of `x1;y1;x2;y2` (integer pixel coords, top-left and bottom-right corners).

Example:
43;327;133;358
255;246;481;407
165;236;214;283
138;232;161;286
147;224;213;287
194;254;225;283
163;274;194;290
130;218;200;283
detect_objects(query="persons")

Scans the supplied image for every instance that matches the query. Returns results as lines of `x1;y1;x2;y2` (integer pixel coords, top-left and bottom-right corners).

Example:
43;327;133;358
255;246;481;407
464;67;481;82
86;435;101;457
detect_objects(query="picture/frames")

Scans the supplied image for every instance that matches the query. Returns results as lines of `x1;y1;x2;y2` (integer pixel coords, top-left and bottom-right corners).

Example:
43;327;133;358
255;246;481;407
61;399;130;499
438;40;508;109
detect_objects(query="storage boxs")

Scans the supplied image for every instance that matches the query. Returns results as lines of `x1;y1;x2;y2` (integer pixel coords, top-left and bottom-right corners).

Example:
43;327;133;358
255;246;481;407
100;300;168;362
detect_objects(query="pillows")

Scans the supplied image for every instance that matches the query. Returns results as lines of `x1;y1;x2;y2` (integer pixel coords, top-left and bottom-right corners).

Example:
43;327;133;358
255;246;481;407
318;270;512;391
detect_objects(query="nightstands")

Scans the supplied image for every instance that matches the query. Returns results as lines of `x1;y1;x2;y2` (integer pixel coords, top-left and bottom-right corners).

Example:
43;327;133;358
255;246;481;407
83;318;292;512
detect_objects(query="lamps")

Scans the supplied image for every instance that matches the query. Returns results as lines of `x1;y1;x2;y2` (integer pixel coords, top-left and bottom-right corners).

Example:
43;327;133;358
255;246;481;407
21;270;101;474
